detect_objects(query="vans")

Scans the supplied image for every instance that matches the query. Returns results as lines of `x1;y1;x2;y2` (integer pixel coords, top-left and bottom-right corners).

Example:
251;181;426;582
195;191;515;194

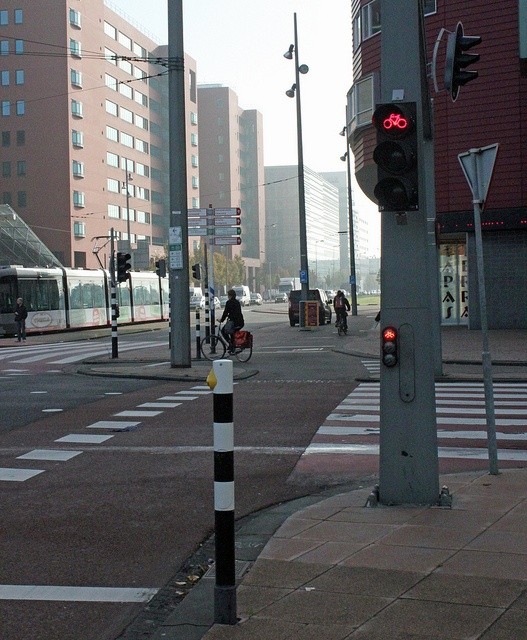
289;288;332;326
230;286;250;307
189;286;202;296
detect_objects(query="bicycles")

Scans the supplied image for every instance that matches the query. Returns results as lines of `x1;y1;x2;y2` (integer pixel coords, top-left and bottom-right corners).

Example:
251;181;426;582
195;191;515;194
202;320;252;363
336;310;348;336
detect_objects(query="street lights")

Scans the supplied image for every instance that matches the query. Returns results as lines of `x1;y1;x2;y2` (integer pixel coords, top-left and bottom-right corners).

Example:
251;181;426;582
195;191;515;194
315;239;324;288
121;173;134;253
339;124;358;317
285;13;310;331
264;223;276;301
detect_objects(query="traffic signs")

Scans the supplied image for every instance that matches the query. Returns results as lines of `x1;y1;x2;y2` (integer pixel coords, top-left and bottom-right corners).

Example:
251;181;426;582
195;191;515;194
187;228;208;236
187;209;206;217
187;219;207;226
214;218;240;225
214;208;240;216
214;228;241;236
169;227;183;269
214;237;241;245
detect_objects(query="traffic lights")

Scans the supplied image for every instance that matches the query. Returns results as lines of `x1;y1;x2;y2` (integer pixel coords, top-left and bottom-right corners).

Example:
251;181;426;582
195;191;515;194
375;103;418;213
445;21;483;101
156;260;166;277
382;328;398;366
192;264;200;280
117;253;130;283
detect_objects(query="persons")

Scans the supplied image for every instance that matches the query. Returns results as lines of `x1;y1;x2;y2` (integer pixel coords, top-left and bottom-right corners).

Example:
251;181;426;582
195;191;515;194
14;297;28;342
334;290;350;329
216;289;244;353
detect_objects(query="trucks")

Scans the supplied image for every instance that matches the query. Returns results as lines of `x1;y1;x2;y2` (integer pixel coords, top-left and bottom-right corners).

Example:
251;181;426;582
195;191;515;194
278;278;302;299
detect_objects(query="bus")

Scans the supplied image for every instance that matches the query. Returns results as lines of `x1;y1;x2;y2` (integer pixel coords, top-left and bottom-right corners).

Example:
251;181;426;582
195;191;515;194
0;264;169;335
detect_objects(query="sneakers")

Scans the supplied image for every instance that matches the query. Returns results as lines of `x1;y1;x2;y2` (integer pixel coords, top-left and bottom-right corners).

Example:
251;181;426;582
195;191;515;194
227;341;233;349
23;340;26;342
16;339;20;342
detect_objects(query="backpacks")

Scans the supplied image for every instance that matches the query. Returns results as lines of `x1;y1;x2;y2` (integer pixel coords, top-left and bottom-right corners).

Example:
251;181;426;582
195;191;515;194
335;296;343;308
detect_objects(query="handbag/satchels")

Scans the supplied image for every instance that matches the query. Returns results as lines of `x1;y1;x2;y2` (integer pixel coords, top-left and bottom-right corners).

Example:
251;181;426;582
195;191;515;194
234;330;250;346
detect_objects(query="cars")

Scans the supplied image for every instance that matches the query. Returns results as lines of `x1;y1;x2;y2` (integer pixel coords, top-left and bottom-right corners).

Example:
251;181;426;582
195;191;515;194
325;290;335;302
214;297;220;309
189;295;205;309
251;293;262;305
220;296;229;308
275;293;287;303
